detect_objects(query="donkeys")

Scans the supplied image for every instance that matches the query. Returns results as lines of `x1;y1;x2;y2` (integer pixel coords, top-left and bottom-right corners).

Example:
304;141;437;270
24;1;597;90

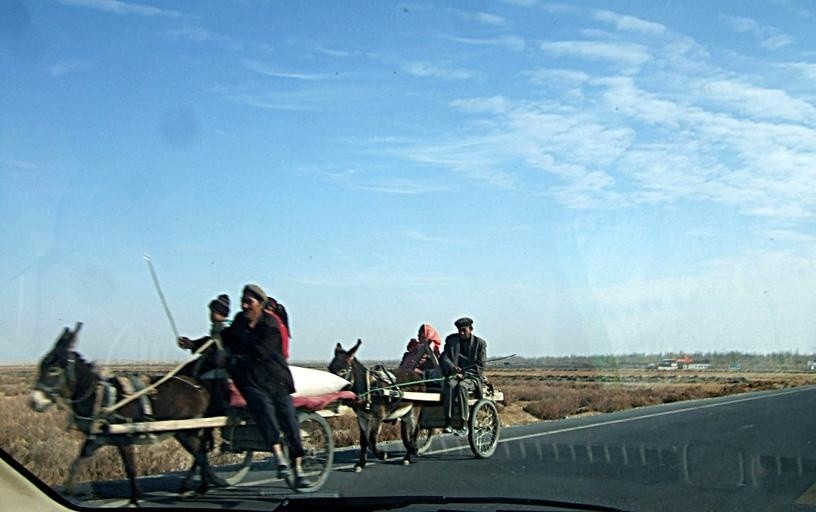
324;338;427;473
26;322;211;508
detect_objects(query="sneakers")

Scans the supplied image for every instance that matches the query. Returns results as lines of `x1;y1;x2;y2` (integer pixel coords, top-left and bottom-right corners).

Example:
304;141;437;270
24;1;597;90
443;425;468;437
279;463;309;489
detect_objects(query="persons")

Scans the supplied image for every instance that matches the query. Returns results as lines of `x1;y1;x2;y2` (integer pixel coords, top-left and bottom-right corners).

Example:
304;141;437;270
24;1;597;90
439;317;488;436
263;296;292;360
403;323;443;387
399;338;419;367
178;284;311;488
191;294;234;378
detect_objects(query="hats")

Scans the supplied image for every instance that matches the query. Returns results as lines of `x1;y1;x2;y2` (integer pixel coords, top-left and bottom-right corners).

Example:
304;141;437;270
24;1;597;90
455;317;472;328
210;293;230;318
244;284;266;303
